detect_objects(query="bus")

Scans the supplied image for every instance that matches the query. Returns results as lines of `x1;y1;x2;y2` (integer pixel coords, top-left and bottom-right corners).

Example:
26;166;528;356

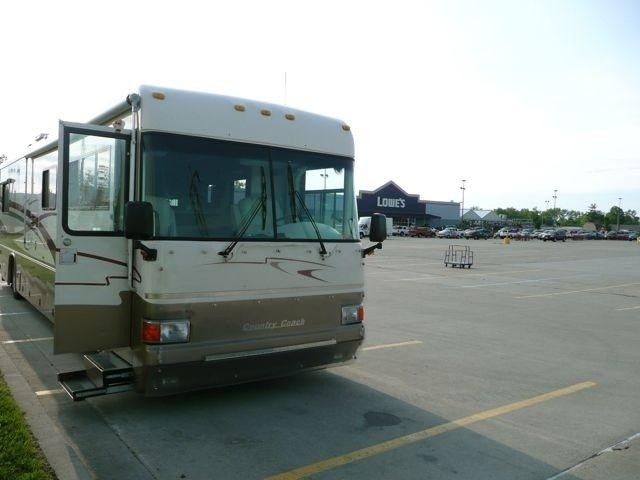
0;85;387;403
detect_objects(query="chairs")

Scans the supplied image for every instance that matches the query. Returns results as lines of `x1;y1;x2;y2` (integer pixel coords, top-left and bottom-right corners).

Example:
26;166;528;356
232;171;285;232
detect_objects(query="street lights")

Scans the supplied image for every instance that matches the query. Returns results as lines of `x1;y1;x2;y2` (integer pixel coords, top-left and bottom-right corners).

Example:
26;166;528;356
319;173;328;191
544;199;550;224
552;189;558;224
460;180;465;223
618;197;621;226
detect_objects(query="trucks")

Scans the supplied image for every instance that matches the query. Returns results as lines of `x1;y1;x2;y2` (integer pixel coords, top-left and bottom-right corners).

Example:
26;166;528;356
358;217;394;241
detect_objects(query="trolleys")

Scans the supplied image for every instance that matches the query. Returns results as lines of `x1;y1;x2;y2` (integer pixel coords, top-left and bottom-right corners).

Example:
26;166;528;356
443;245;474;269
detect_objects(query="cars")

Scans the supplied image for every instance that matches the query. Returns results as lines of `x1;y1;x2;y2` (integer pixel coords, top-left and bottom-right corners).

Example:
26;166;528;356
392;225;640;242
